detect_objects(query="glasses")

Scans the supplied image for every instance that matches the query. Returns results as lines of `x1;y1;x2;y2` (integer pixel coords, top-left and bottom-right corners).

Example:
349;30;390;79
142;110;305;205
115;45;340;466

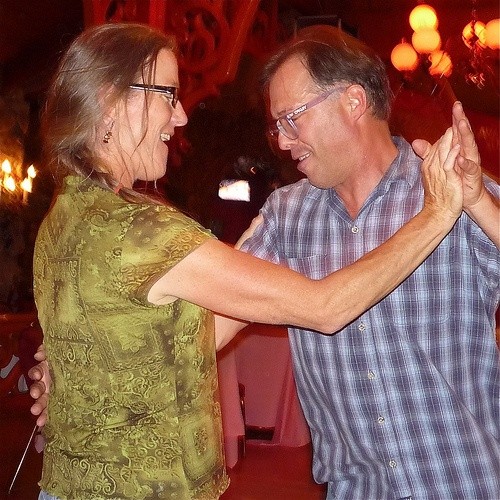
268;86;349;139
128;83;181;108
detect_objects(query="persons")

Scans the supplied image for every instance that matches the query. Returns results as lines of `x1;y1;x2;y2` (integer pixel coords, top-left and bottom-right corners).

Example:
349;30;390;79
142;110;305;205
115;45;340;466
28;20;500;500
31;14;468;500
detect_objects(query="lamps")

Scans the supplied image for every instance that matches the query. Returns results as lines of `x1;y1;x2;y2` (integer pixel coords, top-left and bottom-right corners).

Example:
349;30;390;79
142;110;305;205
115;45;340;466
461;6;500;90
1;157;38;206
389;2;453;100
